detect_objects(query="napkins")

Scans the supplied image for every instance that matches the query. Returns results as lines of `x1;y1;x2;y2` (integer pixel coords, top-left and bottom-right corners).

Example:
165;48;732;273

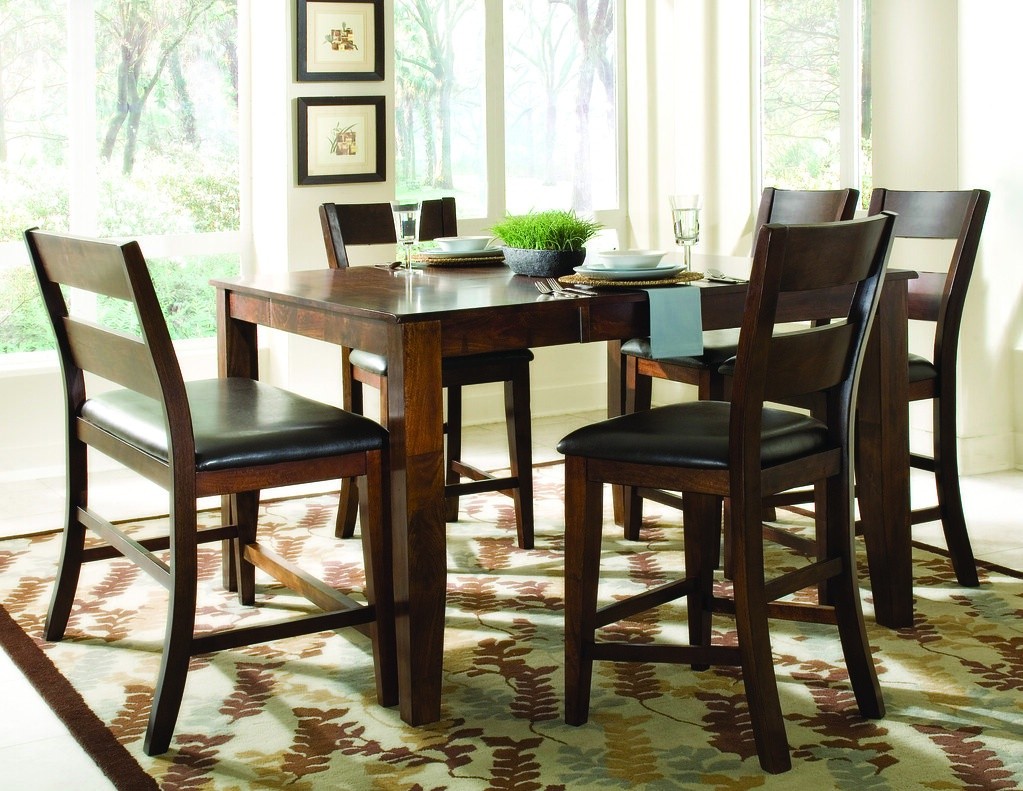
628;285;705;360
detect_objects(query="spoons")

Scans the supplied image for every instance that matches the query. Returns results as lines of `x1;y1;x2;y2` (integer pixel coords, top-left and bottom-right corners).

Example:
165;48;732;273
707;268;750;282
375;261;402;269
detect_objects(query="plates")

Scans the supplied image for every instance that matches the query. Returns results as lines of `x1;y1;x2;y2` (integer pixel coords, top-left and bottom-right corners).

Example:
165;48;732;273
557;273;706;286
407;257;505;265
422;247;503;257
573;265;686;279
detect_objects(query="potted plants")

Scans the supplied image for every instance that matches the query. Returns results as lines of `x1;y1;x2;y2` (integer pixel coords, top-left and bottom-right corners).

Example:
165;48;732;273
476;208;606;278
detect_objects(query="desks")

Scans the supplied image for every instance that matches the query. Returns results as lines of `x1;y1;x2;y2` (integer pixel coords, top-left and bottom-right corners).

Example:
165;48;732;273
205;258;920;729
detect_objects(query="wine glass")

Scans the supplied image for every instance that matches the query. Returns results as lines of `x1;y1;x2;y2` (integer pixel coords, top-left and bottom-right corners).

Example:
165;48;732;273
390;199;422;272
669;194;701;271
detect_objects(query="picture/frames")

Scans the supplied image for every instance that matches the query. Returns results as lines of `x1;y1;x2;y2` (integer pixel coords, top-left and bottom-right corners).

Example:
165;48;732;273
296;96;387;187
296;0;385;83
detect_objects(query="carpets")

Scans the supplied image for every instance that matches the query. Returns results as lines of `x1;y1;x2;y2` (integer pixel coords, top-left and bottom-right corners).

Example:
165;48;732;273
0;458;1023;791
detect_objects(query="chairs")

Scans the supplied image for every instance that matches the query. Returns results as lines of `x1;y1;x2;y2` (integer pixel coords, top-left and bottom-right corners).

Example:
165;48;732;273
714;186;996;629
21;225;395;757
558;209;902;774
616;188;864;570
319;197;537;553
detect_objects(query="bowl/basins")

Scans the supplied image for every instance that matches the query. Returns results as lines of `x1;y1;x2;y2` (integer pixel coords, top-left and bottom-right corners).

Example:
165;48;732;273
596;250;669;268
433;236;493;249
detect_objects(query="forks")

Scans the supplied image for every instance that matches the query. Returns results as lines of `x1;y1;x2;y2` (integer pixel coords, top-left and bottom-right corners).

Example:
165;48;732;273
534;281;578;299
546;278;598;295
